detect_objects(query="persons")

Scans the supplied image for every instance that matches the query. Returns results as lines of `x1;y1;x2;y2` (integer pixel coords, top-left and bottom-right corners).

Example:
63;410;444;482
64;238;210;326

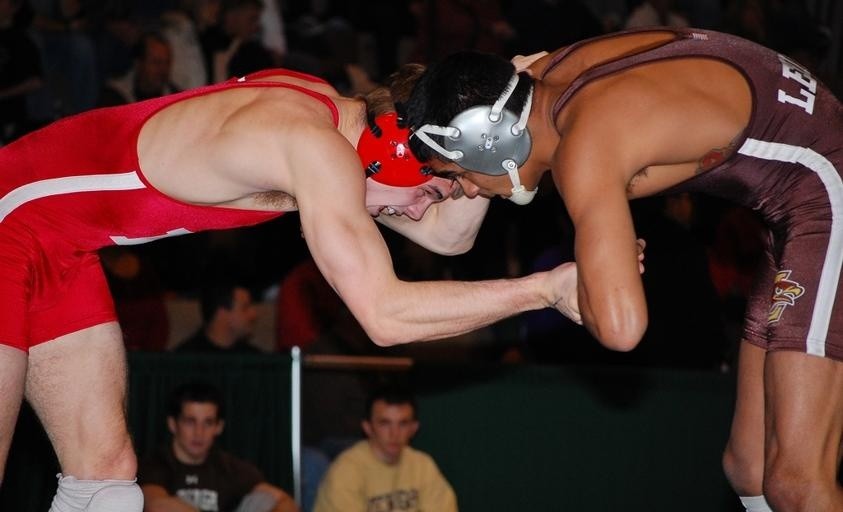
393;24;842;512
133;384;301;511
0;2;843;359
0;48;647;511
312;383;460;511
